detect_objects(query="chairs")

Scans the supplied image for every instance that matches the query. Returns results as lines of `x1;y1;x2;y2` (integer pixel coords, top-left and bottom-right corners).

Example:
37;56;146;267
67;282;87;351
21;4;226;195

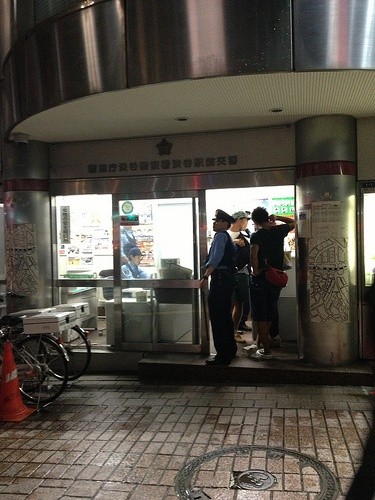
99;268;132;300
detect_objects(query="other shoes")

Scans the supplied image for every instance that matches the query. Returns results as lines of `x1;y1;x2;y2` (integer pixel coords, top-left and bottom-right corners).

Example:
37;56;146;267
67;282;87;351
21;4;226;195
234;333;246;344
240;321;252;331
266;339;282;348
242;345;257;355
253;352;273;359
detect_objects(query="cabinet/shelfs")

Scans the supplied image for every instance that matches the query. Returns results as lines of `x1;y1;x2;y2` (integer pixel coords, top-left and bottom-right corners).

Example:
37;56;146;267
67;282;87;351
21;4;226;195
65;287;97;342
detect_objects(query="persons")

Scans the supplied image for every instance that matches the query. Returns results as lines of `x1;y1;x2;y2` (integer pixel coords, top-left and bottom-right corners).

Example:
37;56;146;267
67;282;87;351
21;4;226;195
250;207;297;357
120;225;139;266
229;211;279;350
199;209;239;367
120;248;149;280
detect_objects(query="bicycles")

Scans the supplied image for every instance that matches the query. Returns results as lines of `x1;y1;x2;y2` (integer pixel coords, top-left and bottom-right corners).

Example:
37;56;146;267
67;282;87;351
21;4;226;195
0;315;70;411
0;291;92;381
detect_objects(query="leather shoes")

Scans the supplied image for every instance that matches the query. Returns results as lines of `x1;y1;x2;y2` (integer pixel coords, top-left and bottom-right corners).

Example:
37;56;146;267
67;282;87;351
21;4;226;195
205;356;231;366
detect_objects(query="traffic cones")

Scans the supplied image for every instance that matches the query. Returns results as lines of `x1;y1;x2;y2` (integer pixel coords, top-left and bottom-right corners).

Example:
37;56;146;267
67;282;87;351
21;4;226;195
0;341;38;422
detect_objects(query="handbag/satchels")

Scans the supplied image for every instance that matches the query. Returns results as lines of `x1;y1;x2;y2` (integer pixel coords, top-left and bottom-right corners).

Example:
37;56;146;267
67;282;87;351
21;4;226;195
235;233;252;274
265;267;288;288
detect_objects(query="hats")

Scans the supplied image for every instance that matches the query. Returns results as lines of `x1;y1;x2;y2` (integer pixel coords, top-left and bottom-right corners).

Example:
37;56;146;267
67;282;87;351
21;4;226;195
212;209;234;223
129;248;143;256
231;211;253;220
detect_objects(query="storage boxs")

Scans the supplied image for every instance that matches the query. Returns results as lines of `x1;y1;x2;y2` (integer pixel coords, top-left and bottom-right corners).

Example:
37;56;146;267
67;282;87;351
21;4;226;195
55;302;90;319
24;312;75;334
11;308;55;318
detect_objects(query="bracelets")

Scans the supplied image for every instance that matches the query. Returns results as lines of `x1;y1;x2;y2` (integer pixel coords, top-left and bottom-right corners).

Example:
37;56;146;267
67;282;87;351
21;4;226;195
201;274;208;280
